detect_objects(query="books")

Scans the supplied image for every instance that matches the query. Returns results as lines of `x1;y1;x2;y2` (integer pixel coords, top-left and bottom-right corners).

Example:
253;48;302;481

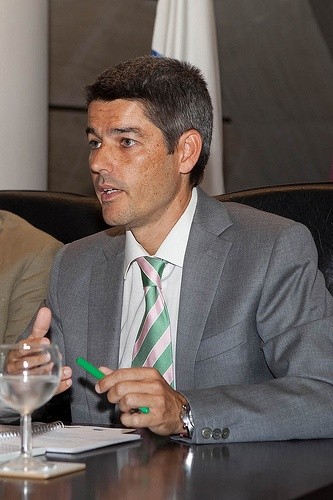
0;421;142;463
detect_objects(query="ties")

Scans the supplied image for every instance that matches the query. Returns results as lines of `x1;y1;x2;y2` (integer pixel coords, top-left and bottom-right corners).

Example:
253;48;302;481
130;256;174;392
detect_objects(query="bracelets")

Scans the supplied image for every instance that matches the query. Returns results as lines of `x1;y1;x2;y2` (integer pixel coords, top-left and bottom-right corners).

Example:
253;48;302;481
179;403;194;438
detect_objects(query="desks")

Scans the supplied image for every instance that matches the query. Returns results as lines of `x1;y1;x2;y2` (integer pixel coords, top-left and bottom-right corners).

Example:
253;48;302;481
0;425;333;500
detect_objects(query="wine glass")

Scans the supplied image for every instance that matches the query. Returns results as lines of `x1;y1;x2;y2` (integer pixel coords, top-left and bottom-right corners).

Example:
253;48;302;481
0;345;62;475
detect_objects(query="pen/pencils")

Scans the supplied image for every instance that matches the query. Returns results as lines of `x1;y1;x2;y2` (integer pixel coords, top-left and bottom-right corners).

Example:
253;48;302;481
77;358;149;414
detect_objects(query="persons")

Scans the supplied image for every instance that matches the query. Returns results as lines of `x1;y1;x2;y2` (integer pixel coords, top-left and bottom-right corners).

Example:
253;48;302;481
0;57;333;444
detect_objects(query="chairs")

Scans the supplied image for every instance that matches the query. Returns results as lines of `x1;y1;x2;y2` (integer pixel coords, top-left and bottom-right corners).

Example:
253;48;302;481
212;181;333;297
0;189;115;246
